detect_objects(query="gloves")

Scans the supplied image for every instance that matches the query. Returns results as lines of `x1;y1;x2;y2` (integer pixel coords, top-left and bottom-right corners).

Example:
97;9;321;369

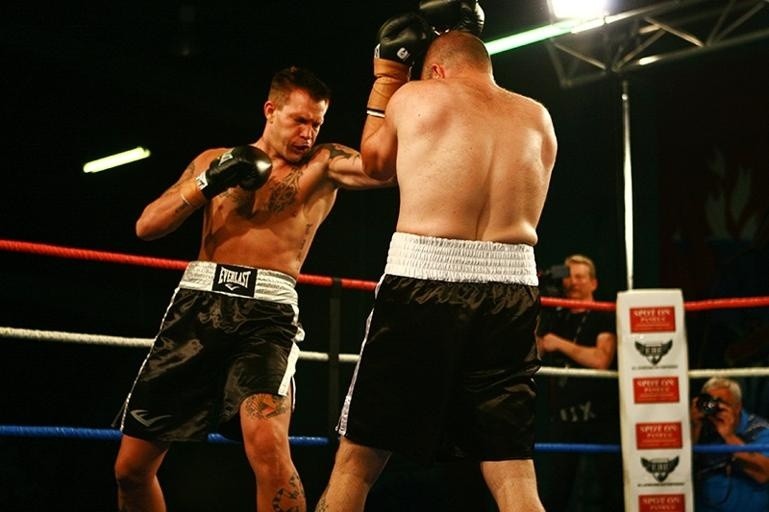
365;11;433;118
178;143;273;213
418;0;486;38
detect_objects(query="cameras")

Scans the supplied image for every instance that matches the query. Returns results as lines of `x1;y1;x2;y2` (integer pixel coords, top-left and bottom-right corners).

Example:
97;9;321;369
696;392;722;416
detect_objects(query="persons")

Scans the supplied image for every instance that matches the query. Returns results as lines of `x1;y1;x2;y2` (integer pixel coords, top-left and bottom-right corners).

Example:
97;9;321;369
110;67;397;512
533;254;616;423
313;13;558;512
690;378;769;512
